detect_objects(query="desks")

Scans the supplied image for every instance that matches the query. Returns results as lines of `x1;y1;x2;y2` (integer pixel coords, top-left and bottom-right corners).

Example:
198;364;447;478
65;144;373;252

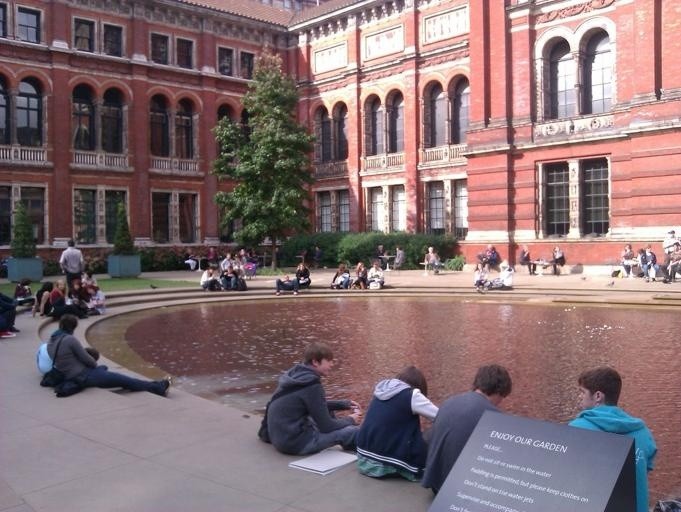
623;259;640;278
294;255;304;264
377;255;397;272
529;260;550;275
192;256;206;272
258;255;272;268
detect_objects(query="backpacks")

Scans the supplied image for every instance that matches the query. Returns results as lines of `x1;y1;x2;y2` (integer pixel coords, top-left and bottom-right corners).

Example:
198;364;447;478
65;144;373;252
35;340;57;372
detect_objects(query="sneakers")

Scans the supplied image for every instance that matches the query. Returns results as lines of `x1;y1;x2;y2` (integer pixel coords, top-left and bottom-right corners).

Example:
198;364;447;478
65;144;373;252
1;329;17;340
162;375;172;396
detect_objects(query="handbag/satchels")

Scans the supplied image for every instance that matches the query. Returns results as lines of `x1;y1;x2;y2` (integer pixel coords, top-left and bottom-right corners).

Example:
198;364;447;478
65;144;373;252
39;366;65;385
256;379;321;446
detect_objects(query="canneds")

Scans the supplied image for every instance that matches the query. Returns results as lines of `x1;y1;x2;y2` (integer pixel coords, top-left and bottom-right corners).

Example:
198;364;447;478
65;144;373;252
351;406;360;414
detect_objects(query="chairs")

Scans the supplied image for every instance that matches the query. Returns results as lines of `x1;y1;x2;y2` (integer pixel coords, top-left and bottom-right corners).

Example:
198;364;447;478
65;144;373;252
425;253;443;276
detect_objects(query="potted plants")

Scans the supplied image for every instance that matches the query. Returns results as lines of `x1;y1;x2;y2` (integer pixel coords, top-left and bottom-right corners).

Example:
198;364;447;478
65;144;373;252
7;199;44;282
107;193;141;278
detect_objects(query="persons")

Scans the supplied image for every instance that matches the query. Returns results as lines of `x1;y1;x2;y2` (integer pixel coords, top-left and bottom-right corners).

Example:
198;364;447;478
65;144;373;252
258;342;359;454
45;313;175;399
0;239;106;338
356;365;439;482
566;363;658;512
421;363;514;497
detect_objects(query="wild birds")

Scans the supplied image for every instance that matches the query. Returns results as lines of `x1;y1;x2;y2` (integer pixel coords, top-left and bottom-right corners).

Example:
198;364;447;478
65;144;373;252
150;284;157;289
